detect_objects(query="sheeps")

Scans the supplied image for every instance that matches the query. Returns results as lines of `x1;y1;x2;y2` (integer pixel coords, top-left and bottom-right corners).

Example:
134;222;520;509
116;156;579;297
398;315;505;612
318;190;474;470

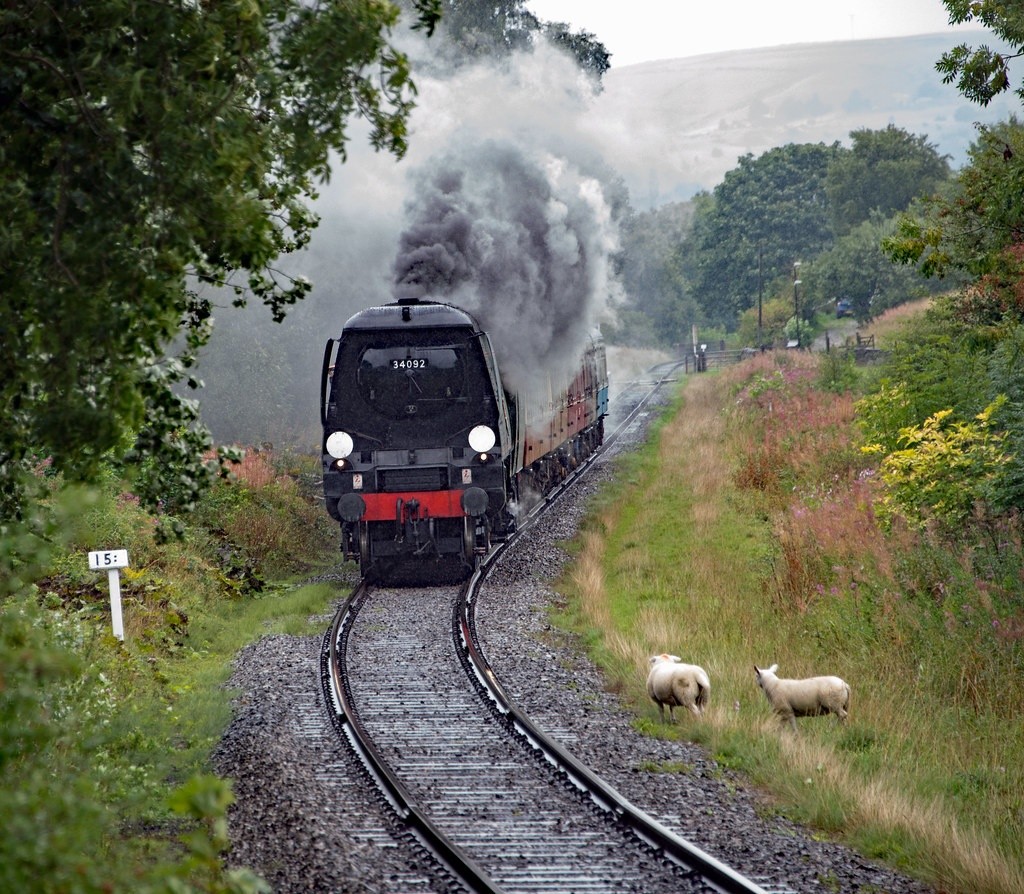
645;653;711;726
753;663;852;733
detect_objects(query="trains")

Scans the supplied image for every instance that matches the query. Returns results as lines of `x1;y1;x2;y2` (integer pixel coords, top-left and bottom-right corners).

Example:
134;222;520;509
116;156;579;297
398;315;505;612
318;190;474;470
319;296;609;576
